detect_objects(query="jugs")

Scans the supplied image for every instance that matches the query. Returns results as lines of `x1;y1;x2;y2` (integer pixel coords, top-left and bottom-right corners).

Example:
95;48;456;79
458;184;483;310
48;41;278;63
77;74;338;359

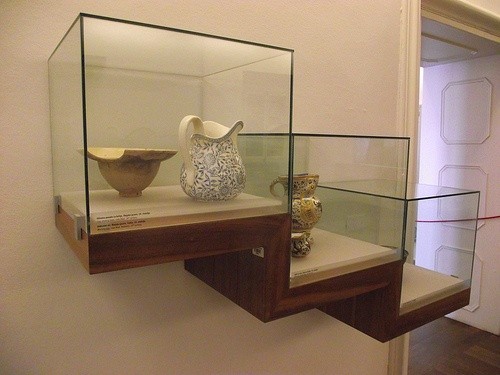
179;114;245;201
291;230;311;257
270;174;323;232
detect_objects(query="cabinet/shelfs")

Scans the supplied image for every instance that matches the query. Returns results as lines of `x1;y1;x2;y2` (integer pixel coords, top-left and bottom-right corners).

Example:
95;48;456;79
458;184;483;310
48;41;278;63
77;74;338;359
52;186;470;344
